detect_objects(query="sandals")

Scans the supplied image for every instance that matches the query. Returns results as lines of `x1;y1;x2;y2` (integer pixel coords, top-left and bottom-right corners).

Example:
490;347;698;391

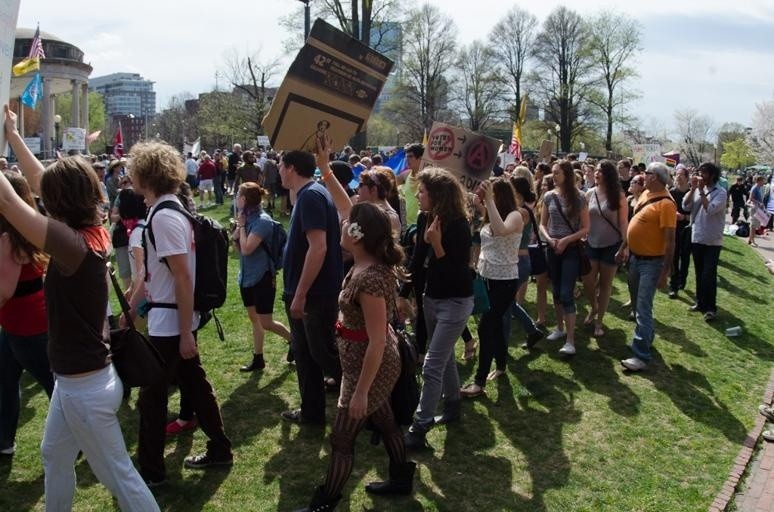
459;382;485;398
533;311;604;337
460;338;479;360
486;368;506;381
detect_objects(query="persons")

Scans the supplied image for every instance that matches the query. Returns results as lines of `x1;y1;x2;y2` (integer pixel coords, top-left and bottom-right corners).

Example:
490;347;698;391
0;99;164;510
117;137;235;490
1;136;774;458
302;202;418;511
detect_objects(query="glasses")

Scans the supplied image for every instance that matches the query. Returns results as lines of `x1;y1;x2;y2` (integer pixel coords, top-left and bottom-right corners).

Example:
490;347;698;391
358;182;367;188
629;180;637;184
119;180;127;185
644;170;652;174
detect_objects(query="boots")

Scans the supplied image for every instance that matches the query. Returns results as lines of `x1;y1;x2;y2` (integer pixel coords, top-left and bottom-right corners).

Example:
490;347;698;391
289;485;343;512
364;462;416;495
434;399;461;424
239;354;265;371
287;344;294;362
402;426;429;448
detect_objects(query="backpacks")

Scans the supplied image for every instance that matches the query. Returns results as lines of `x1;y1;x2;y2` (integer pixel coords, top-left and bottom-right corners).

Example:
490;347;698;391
148;200;230;311
268;220;287;270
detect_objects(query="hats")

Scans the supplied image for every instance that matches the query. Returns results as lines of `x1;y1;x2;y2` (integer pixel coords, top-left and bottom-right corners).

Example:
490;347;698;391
331;159;353;185
108;159;127;170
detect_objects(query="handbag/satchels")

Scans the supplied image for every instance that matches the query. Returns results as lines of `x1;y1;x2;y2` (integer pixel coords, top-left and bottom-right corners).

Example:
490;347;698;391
577;240;592;267
112;221;129;248
110;326;165;388
528;243;547;275
396;329;419;370
367;330;420;430
678;224;691;256
470;276;491;314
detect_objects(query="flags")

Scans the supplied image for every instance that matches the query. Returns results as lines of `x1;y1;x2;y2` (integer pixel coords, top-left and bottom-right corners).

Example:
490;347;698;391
112;125;125;158
13;56;40;78
508;95;527;158
18;70;44;111
28;25;46;60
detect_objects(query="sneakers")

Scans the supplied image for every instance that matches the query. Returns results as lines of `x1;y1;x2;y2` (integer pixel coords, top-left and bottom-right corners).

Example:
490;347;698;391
620;357;648;371
164;419;193;434
546;329;563;341
184;452;233;468
1;442;18;454
520;329;544;349
558;343;576;355
281;408;308;422
669;281;716;321
